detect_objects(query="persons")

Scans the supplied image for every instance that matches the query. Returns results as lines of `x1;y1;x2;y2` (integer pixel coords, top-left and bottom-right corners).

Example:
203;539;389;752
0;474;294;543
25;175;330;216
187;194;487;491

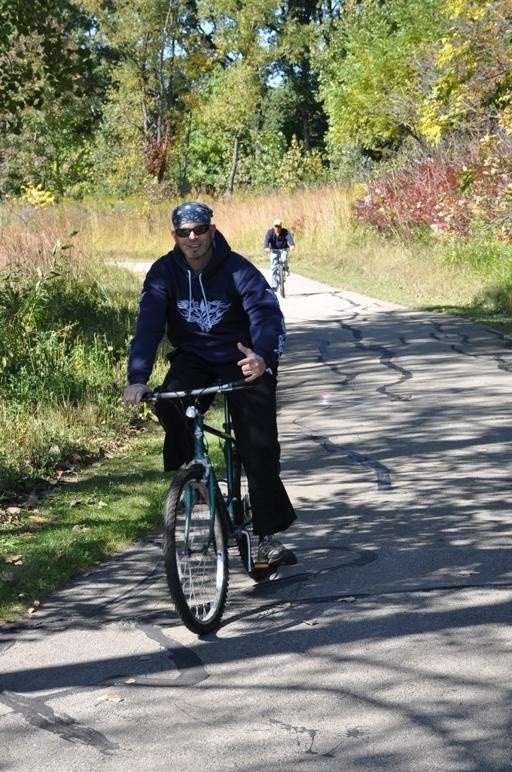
122;200;299;568
262;218;294;290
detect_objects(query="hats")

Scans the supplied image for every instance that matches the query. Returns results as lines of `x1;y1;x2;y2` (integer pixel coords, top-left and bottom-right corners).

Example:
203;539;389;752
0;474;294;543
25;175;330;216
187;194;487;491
274;219;282;226
170;201;213;227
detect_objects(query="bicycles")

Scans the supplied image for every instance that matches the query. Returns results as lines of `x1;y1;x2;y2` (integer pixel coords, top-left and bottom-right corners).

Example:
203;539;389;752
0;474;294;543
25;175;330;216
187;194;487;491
270;247;290;298
140;367;273;636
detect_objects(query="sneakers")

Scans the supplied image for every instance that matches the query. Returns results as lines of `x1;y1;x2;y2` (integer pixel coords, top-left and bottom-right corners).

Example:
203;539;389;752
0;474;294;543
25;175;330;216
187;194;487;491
256;538;298;567
176;487;198;515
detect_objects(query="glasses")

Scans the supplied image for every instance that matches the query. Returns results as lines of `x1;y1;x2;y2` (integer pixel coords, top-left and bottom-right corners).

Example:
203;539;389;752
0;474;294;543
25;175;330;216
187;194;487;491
275;225;281;228
174;225;209;238
